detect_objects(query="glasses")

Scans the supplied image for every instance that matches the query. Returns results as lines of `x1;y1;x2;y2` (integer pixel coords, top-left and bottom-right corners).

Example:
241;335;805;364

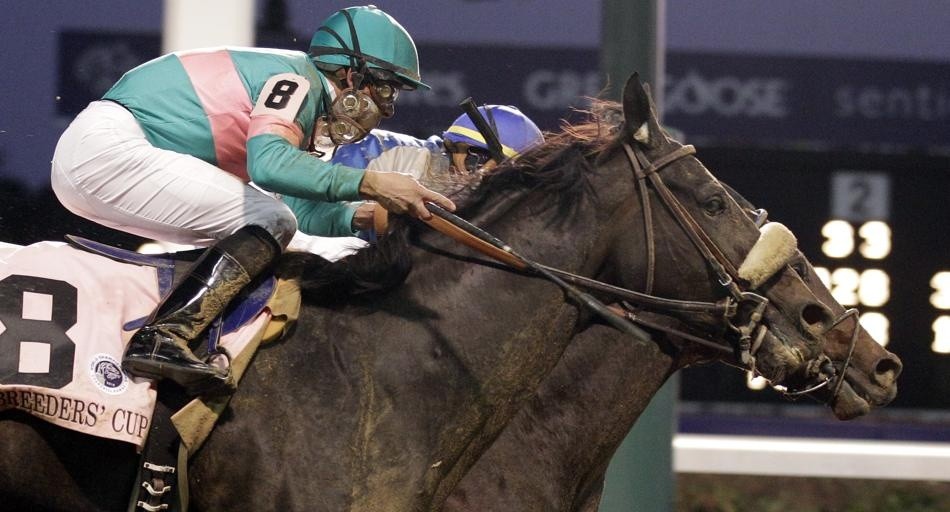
351;67;399;100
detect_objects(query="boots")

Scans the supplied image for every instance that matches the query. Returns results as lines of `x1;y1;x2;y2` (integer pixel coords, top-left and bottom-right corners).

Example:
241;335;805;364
119;242;252;386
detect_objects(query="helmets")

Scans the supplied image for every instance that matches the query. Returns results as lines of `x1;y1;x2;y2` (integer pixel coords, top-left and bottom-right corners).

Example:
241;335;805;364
441;101;548;164
307;3;433;93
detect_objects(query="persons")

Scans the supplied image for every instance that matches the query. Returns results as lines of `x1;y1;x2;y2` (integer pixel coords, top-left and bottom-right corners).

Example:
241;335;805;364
49;3;456;397
248;103;545;263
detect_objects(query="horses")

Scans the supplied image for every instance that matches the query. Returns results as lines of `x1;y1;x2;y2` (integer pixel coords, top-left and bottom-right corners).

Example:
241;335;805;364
0;69;904;512
